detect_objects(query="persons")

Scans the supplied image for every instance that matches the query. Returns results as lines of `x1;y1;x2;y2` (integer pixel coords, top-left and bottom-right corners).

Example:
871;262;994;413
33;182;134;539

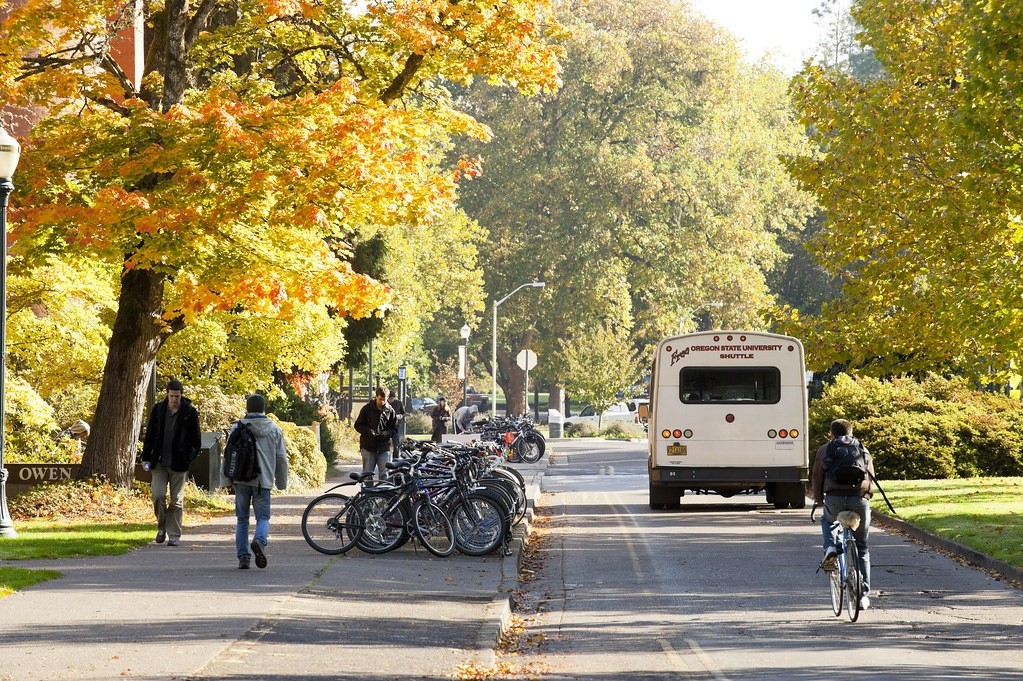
431;398;451;445
354;387;399;488
452;405;478;435
221;396;288;570
812;419;875;611
54;419;90;452
142;381;202;547
388;391;405;463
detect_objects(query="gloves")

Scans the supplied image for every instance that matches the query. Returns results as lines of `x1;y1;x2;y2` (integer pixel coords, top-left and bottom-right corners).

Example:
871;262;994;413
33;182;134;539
377;431;387;441
368;429;376;439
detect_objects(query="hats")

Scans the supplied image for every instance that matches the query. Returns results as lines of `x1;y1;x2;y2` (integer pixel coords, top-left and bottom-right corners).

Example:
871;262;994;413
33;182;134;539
247;394;265;413
390;391;395;397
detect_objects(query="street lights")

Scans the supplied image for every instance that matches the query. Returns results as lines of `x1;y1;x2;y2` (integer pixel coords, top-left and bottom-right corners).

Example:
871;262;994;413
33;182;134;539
678;303;724;335
0;126;22;537
461;323;471;406
493;283;545;418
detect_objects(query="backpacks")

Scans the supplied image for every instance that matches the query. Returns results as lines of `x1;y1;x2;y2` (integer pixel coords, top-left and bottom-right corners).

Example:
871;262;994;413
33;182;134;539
823;437;868;485
223;419;261;482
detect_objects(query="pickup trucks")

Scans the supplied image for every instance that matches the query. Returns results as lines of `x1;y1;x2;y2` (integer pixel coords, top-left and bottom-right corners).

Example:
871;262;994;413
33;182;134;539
461;387;488;412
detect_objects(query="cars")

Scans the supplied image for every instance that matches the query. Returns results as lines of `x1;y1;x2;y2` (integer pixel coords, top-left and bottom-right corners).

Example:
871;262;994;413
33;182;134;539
401;398;437;415
562;400;649;432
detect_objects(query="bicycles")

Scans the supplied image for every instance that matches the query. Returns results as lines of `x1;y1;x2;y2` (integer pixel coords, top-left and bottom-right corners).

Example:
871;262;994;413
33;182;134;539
479;414;546;464
810;499;865;622
300;437;528;559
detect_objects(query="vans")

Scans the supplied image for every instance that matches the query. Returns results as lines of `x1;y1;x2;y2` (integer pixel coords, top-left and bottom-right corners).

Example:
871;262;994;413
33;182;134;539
637;330;819;512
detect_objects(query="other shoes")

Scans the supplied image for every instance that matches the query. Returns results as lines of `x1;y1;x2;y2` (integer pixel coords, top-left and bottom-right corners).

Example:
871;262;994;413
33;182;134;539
168;535;179;546
859;595;870;610
251;541;267;569
156;529;166;543
238;556;251;569
820;545;837;567
